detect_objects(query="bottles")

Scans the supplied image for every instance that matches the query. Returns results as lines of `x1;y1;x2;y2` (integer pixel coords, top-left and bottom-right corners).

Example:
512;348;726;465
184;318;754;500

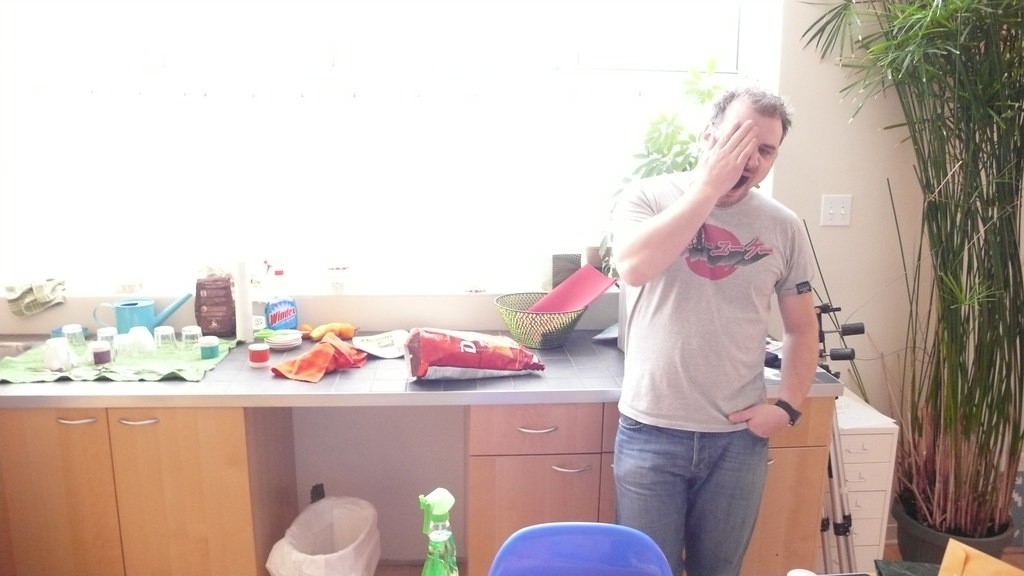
586;247;602;273
264;270;297;330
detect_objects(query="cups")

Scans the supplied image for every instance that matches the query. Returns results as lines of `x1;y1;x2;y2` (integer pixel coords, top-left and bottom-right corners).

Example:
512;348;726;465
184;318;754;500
113;334;142;364
63;325;87;352
181;326;202;350
154;326;177;352
128;326;154;352
91;341;111;364
97;327;117;348
249;344;270;368
198;336;219;359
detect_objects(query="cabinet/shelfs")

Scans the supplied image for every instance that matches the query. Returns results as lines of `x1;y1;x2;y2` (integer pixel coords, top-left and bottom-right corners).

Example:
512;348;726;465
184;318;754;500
0;330;843;576
817;385;899;575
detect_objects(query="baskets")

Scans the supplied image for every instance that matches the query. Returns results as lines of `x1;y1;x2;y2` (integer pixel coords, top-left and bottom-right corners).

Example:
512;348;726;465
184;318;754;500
494;292;587;350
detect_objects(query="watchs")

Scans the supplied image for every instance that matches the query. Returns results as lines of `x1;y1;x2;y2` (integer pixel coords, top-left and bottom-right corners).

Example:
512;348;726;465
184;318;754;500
775;398;802;426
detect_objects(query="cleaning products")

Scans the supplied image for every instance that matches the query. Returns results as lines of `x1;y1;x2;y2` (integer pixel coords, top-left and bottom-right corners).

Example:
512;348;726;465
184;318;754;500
419;487;460;576
265;269;299;329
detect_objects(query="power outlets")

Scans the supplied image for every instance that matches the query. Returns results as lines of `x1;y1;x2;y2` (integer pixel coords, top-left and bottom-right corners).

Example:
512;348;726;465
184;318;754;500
820;194;851;225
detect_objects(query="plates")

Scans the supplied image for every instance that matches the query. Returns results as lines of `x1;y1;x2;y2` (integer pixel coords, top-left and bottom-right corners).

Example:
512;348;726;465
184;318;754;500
265;329;302;350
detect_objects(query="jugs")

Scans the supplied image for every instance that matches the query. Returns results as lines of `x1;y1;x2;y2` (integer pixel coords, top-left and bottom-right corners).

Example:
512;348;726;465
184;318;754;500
93;293;192;336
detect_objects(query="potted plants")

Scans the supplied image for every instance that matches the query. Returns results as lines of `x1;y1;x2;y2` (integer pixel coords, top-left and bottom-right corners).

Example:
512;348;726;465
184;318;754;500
799;1;1024;563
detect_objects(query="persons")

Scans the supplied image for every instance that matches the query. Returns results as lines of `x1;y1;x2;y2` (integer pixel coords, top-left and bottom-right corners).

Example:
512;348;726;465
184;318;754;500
609;83;819;576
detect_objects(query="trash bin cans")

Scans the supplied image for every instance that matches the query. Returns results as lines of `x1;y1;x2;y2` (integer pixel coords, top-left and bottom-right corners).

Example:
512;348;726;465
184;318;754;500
285;496;378;576
